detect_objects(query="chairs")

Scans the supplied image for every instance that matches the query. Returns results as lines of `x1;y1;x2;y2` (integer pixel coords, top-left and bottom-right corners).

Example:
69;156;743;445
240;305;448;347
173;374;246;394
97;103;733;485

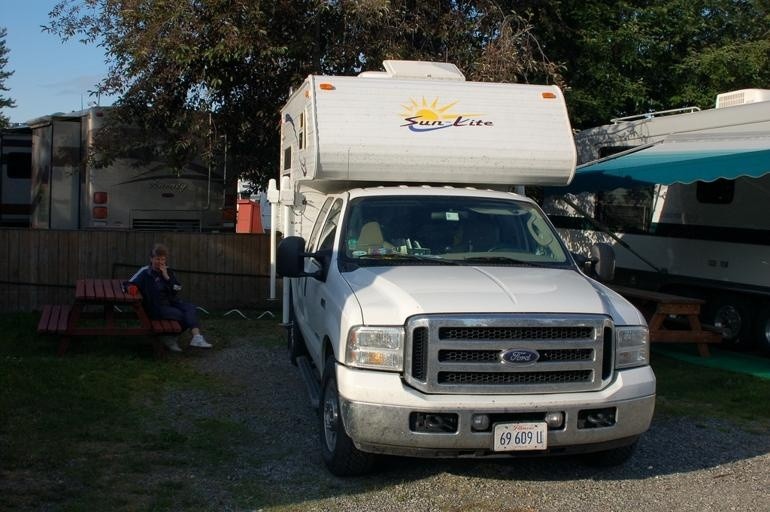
456;226;496;252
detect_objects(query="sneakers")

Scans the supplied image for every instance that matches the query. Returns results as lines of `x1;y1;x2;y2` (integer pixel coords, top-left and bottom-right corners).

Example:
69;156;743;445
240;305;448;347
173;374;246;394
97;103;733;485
189;335;213;348
161;336;182;352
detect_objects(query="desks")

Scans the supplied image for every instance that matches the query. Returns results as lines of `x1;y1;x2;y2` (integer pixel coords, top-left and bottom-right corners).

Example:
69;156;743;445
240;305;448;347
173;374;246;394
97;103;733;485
54;278;167;359
604;283;712;359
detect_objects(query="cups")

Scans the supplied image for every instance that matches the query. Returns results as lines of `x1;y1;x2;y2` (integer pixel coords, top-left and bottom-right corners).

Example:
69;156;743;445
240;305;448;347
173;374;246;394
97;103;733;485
128;285;137;297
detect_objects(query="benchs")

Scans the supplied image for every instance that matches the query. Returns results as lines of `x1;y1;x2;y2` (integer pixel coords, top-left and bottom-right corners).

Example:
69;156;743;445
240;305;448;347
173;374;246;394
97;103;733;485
37;303;71;335
150;319;182;333
648;312;729;334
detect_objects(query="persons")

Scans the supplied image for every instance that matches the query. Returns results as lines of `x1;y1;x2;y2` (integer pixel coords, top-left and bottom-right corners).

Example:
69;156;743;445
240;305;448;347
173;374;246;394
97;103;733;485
123;242;213;353
